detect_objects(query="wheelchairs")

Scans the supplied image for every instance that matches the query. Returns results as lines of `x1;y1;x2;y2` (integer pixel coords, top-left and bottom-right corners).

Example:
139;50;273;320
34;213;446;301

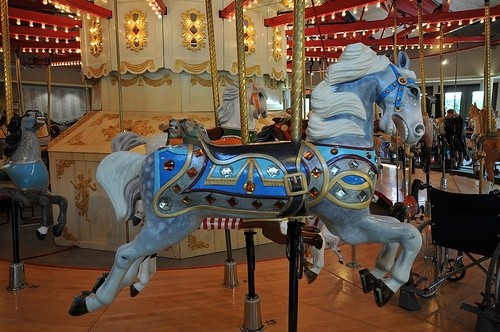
410;184;500;308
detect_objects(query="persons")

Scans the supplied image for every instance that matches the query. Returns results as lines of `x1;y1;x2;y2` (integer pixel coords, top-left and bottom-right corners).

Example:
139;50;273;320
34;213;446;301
444;109;463;169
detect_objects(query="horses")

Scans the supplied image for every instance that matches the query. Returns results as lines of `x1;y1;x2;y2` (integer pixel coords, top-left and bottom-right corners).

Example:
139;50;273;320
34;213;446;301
66;41;427;317
467;102;500;189
0;112;69;241
